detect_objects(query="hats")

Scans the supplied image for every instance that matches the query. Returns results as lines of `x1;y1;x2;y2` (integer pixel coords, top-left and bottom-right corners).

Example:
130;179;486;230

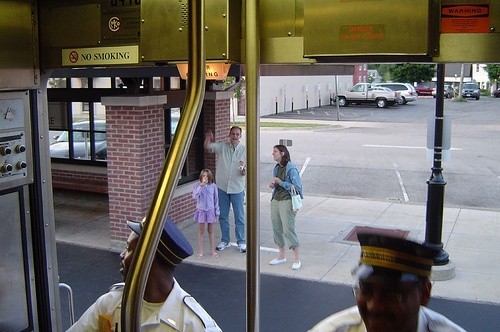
126;213;194;266
350;232;435;280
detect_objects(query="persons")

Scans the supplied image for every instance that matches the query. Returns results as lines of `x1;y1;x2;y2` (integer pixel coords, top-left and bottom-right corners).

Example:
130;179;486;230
268;144;304;270
191;169;220;259
305;232;467;332
65;215;223;332
203;126;247;253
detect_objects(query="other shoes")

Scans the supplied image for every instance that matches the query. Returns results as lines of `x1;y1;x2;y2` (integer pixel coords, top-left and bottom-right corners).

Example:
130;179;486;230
268;256;287;265
196;251;205;259
291;260;302;269
209;250;219;258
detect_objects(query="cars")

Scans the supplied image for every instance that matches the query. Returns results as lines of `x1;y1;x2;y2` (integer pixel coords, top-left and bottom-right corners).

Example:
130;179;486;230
493;87;500;98
414;82;435;96
431;85;457;99
48;118;107;161
461;82;480;100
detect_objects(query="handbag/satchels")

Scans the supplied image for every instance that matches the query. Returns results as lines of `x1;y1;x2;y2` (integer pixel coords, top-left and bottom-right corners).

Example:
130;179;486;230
291;184;303;212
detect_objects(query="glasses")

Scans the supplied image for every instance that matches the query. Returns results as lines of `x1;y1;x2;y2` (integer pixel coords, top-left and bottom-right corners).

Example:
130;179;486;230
352;286;418;304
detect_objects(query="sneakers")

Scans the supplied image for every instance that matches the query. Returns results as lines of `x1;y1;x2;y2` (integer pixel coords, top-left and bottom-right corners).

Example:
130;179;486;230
237;243;247;253
216;241;231;251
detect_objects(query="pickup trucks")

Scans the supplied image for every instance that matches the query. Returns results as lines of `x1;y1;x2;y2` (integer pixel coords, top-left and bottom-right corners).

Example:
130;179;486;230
332;83;401;109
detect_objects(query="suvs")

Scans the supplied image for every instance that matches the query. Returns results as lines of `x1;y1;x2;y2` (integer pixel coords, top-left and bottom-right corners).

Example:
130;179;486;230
371;82;419;105
